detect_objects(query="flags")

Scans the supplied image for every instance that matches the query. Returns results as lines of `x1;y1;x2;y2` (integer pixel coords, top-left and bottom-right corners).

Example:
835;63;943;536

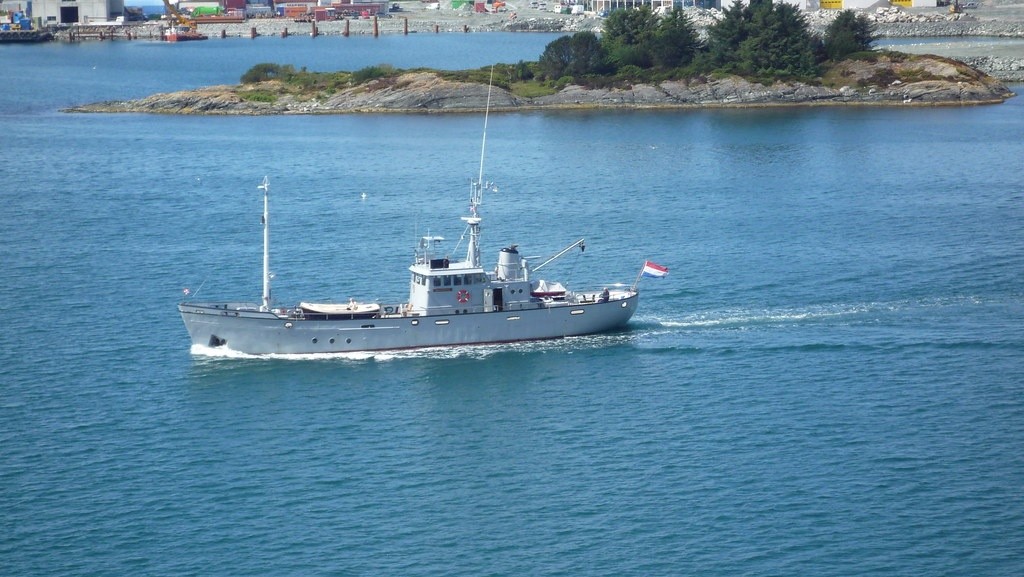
641;261;669;279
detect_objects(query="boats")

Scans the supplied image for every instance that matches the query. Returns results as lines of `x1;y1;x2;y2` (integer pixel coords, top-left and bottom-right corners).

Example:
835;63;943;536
176;63;669;356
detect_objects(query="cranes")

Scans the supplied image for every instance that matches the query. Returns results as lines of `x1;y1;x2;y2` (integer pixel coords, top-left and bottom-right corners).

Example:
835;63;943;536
162;0;197;33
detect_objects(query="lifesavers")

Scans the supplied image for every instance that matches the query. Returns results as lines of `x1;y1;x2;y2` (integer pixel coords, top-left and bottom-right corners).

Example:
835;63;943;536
457;289;470;303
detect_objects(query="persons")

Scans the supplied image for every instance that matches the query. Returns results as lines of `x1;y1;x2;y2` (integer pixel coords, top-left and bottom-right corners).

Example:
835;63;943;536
602;287;610;302
